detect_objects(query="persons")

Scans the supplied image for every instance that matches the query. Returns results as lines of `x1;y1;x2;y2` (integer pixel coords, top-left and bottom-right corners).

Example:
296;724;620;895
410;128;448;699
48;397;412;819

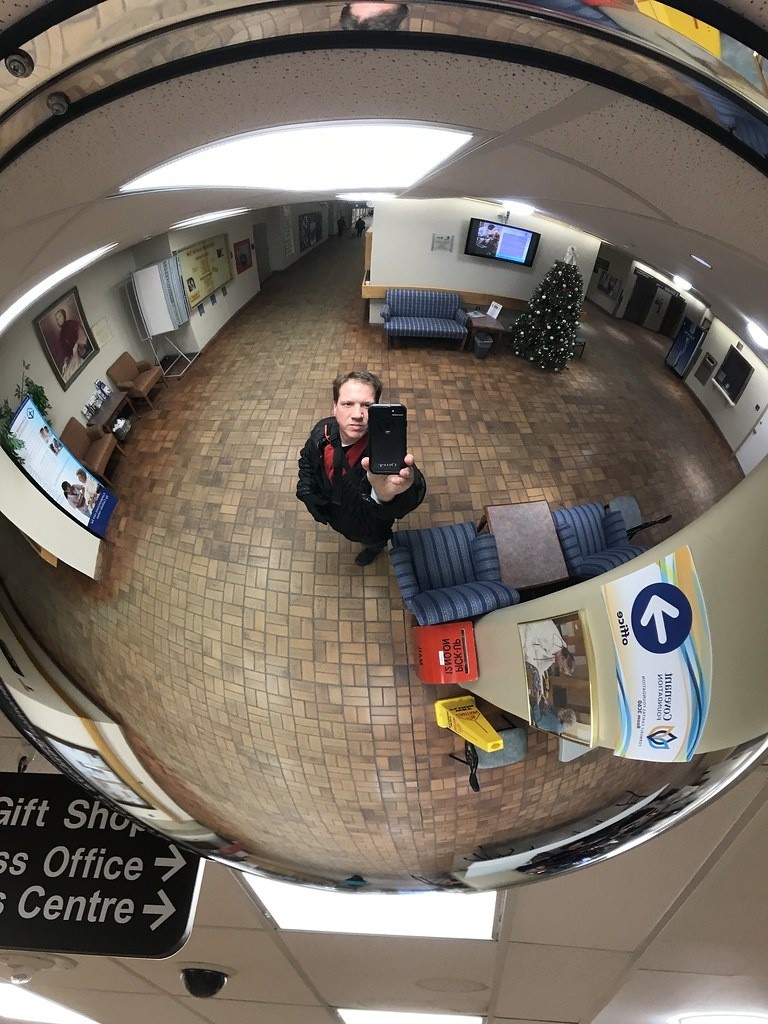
40;426;61;453
518;618;576;735
296;371;427;566
478;225;499;253
355;218;365;236
337;216;346;236
62;469;99;517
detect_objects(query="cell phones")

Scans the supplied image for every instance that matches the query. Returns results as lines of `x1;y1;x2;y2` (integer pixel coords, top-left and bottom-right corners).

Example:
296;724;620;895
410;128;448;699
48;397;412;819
368;404;407;475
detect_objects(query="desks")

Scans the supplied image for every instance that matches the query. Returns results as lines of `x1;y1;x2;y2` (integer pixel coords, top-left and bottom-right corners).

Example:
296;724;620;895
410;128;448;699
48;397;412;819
477;499;569;591
557;737;595;763
465;309;505;356
86;392;142;448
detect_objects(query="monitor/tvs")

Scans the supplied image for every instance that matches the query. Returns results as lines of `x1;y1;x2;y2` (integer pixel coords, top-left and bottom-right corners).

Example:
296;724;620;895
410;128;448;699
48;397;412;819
465;217;541;268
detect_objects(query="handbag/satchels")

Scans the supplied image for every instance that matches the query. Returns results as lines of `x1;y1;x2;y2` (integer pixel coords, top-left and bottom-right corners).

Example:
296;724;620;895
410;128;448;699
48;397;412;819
393;462;428;523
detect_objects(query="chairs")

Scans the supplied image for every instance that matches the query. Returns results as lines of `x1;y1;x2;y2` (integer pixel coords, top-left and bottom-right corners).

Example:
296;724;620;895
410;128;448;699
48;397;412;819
604;495;672;541
551;502;650;582
59;416;127;490
449;714;529;792
106;351;169;410
573;337;587;359
387;522;519;626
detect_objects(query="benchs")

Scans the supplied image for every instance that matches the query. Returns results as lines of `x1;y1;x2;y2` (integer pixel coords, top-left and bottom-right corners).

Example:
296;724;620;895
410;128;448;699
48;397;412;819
379;288;471;352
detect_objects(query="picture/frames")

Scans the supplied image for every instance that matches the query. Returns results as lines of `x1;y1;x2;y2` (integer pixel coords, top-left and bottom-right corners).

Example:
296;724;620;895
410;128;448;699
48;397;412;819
517;607;599;748
32;284;100;392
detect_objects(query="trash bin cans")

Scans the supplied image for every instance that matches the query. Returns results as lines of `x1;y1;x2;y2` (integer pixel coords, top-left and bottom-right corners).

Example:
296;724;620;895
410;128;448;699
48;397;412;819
473;333;493;359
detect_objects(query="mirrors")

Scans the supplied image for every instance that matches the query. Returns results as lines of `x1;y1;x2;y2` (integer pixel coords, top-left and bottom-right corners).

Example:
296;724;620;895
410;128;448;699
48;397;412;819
0;0;768;895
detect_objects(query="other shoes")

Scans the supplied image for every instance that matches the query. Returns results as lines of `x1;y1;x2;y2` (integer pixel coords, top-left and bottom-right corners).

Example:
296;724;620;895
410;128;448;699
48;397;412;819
355;547;385;566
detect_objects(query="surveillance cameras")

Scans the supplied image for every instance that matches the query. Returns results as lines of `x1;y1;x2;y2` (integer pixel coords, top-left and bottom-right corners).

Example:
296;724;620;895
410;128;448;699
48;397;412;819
175;962;237;997
